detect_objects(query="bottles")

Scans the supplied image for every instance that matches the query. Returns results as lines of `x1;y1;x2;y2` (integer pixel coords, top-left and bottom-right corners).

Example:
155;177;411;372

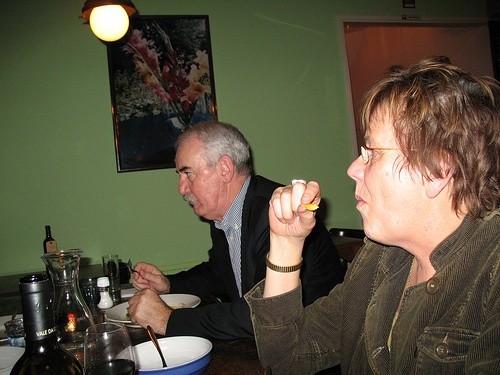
96;276;114;312
42;247;97;371
9;274;85;375
43;225;57;253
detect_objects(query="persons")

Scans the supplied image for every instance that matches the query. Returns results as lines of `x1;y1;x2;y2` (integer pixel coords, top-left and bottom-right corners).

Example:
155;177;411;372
128;120;343;349
244;56;500;375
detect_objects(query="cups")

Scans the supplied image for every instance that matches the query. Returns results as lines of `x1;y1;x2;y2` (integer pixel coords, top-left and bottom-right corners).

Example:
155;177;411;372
101;253;121;303
83;322;135;375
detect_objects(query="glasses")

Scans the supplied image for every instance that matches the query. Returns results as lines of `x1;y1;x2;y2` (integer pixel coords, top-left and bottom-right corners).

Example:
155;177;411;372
361;145;419;165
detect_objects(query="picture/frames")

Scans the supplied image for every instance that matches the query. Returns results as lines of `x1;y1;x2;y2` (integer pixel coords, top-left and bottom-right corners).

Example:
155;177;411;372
107;14;219;173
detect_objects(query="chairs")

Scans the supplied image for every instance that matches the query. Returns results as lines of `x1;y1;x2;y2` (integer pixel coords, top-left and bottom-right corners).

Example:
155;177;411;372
328;227;365;290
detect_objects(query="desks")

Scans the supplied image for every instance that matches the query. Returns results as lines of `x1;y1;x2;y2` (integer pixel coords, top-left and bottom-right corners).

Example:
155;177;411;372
0;286;269;375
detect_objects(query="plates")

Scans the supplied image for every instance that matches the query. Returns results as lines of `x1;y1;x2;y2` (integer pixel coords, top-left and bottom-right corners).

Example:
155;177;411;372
0;346;25;372
104;293;201;329
117;335;212;375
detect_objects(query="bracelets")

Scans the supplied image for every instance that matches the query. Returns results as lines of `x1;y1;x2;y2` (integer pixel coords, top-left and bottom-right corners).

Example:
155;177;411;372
266;251;303;272
